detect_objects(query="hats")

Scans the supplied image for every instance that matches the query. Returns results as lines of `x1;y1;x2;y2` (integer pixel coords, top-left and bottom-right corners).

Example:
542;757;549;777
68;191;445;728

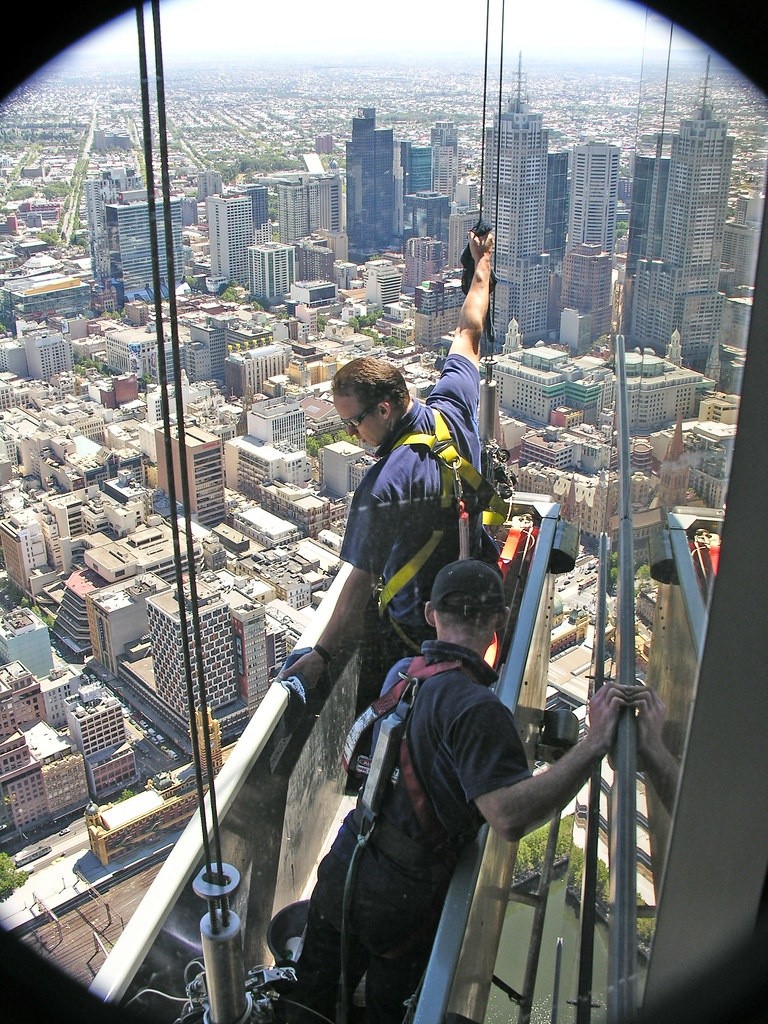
430;555;507;615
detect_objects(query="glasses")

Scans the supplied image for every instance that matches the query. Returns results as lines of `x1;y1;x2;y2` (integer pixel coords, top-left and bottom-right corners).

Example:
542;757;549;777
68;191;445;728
339;402;383;427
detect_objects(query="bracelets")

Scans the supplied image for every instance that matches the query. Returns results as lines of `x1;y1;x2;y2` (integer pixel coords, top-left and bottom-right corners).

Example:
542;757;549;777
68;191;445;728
314;644;331;662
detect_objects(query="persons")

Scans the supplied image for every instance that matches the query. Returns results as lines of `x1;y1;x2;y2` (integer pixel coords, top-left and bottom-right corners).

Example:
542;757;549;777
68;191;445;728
282;556;628;1024
277;230;494;787
629;687;678;818
